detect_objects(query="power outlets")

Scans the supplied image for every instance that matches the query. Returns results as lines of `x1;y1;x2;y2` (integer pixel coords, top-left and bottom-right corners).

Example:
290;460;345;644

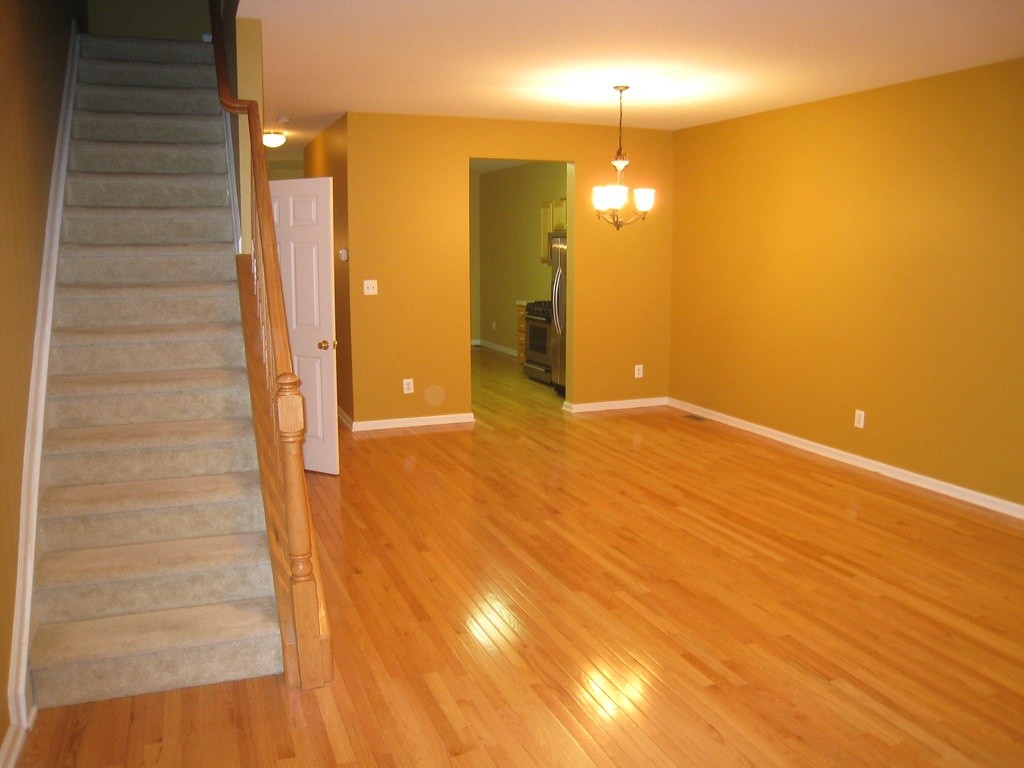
492;322;495;330
403;378;413;394
853;410;864;429
635;364;643;379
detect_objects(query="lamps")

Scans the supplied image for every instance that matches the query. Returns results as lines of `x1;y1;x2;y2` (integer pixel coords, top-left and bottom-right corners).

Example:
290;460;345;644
593;85;656;232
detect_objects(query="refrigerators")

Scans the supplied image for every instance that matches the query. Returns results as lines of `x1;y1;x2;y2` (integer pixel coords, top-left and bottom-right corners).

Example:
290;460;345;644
549;237;567;398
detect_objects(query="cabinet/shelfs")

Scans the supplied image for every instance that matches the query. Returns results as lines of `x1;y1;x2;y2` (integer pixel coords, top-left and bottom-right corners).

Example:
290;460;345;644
524;315;551;385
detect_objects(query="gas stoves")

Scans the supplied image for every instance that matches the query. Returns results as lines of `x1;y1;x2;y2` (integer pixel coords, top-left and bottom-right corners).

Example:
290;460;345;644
526;296;552;319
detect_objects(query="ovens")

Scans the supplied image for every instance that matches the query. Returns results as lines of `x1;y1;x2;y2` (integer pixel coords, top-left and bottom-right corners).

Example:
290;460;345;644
525;313;551;385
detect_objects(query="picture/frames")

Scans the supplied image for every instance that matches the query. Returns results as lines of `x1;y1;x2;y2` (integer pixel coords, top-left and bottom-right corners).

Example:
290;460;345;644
553;199;566;231
540;202;552;262
